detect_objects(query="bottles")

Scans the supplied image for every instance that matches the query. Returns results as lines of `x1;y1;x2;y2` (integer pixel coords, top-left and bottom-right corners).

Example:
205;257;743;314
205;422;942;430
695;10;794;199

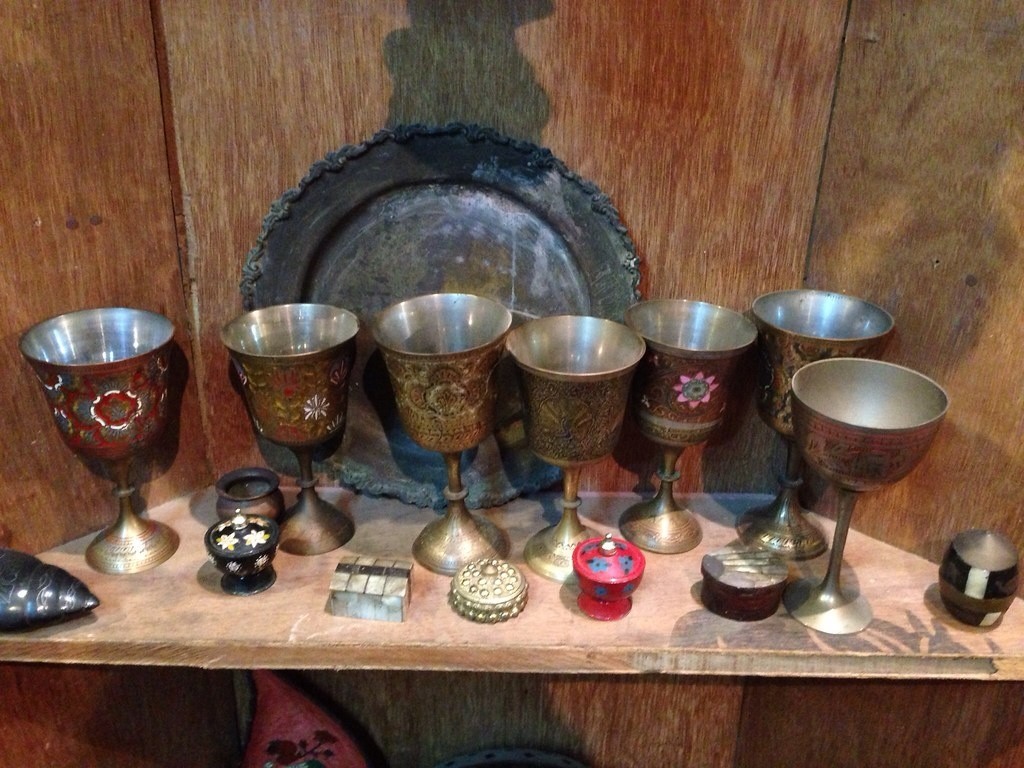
216;467;286;520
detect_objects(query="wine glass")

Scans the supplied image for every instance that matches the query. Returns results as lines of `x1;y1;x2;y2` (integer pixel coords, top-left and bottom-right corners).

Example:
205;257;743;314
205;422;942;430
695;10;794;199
21;307;180;576
620;298;757;554
369;292;514;578
218;303;360;556
736;288;897;560
780;359;948;634
505;316;647;583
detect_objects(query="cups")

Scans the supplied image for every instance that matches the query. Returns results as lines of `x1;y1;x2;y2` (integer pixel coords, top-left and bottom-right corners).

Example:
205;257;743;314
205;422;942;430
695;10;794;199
936;528;1021;629
203;514;281;595
